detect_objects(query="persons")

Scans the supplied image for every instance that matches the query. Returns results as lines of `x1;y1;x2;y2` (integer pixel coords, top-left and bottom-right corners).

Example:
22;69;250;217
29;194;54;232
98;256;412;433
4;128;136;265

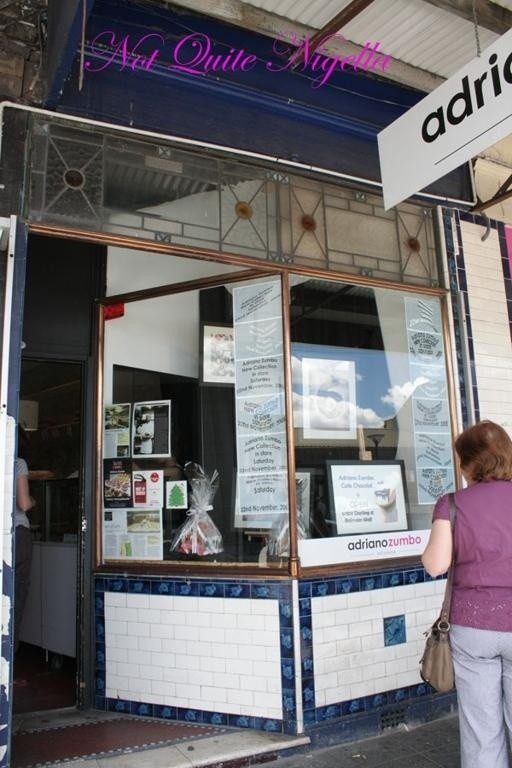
419;419;512;768
137;413;155;454
371;468;397;524
15;421;39;665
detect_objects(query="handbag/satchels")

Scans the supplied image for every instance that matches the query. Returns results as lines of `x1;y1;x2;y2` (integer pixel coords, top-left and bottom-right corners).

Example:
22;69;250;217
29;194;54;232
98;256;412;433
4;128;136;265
418;616;456;695
